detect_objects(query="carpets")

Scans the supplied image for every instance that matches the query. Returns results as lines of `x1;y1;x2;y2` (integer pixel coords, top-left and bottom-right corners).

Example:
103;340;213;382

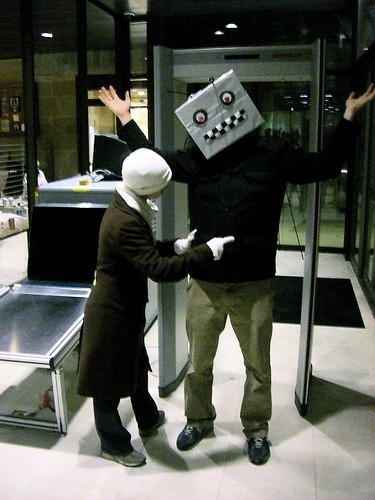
272;274;364;328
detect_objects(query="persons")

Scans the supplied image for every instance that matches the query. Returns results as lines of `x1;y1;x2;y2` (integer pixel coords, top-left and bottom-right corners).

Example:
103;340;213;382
23;161;48;200
77;148;235;467
98;84;375;465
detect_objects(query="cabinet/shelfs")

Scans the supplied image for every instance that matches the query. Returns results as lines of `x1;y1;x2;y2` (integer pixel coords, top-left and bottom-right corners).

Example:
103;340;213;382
0;82;39;139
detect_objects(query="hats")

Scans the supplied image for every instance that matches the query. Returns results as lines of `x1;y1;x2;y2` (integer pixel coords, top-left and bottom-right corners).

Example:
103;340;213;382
121;147;172;195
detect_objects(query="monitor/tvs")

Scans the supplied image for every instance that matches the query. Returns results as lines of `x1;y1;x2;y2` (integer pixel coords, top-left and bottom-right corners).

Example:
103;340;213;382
92;134;129;178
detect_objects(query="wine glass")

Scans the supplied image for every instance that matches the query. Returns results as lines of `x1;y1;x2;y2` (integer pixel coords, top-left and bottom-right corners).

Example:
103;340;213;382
10;97;19;113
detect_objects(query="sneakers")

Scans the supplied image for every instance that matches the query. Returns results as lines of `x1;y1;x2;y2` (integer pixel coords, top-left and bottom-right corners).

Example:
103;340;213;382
248;436;269;465
139;410;165;437
176;424;213;450
102;446;146;467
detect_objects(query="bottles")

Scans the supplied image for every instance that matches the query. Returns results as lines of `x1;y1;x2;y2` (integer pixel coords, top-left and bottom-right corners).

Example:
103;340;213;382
16;203;23;216
0;93;25;133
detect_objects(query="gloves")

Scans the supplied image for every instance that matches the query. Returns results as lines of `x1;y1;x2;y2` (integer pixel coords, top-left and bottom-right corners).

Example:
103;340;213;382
174;228;197;255
206;235;235;261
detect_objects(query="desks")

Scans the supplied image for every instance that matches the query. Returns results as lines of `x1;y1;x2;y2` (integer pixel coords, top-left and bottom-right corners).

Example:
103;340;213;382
32;171;123;288
0;278;95;434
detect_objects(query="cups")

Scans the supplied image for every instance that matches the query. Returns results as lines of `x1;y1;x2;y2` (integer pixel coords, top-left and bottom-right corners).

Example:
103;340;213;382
2;197;14;207
